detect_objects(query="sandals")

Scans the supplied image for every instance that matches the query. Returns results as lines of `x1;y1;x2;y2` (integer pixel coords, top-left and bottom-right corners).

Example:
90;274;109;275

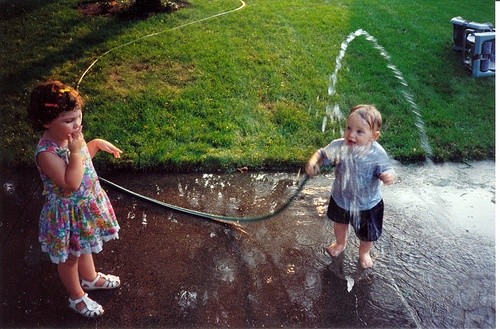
81;271;121;291
68;293;105;318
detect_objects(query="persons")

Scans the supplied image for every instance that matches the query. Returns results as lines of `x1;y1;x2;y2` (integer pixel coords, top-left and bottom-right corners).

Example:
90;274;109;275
305;103;397;269
24;79;121;319
